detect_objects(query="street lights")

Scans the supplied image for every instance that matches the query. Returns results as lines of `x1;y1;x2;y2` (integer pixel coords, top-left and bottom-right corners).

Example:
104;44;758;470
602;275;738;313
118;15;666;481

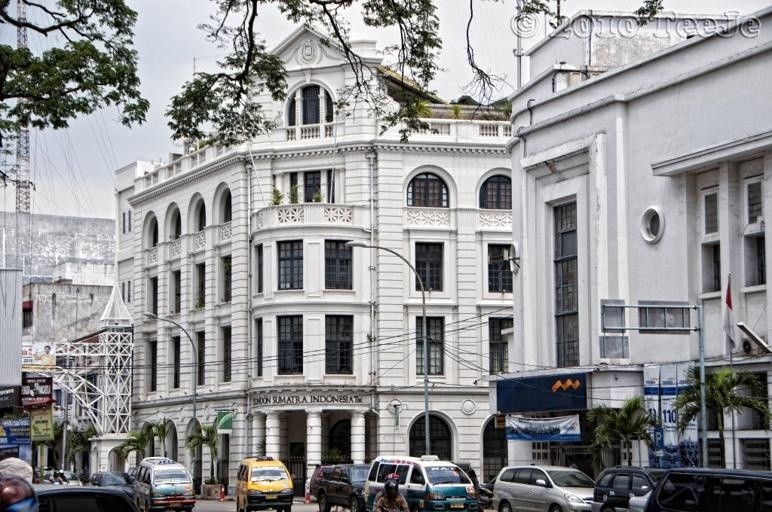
143;311;200;462
736;322;772;354
345;239;432;455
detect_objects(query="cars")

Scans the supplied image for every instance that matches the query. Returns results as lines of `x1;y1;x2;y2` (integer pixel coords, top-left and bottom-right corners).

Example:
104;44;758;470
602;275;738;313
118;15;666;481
479;474;497;490
30;466;140;511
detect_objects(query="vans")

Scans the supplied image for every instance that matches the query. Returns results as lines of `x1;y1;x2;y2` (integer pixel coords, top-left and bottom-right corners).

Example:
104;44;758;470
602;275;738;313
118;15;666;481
364;455;482;511
495;465;598;512
133;456;195;511
234;457;293;512
642;467;771;510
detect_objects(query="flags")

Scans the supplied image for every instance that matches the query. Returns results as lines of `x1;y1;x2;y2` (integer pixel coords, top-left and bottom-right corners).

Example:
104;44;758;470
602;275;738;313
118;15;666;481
722;277;737;356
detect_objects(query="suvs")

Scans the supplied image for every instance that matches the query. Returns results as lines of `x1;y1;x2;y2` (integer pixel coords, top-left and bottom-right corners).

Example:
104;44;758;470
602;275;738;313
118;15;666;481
597;465;665;506
310;464;369;511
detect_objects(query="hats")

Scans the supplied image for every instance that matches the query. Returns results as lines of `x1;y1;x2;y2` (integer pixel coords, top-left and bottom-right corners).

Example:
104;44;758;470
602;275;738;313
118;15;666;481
0;457;33;487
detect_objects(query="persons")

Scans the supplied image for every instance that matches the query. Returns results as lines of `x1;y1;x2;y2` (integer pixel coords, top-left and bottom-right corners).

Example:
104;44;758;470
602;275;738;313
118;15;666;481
372;473;410;512
48;469;64;486
32;345;54;365
568;462;578;470
0;456;41;512
57;470;71;484
466;468;479;489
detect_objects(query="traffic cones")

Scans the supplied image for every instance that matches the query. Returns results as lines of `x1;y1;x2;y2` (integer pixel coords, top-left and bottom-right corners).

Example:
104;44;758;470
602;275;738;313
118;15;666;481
219;485;226;501
305;487;311;505
